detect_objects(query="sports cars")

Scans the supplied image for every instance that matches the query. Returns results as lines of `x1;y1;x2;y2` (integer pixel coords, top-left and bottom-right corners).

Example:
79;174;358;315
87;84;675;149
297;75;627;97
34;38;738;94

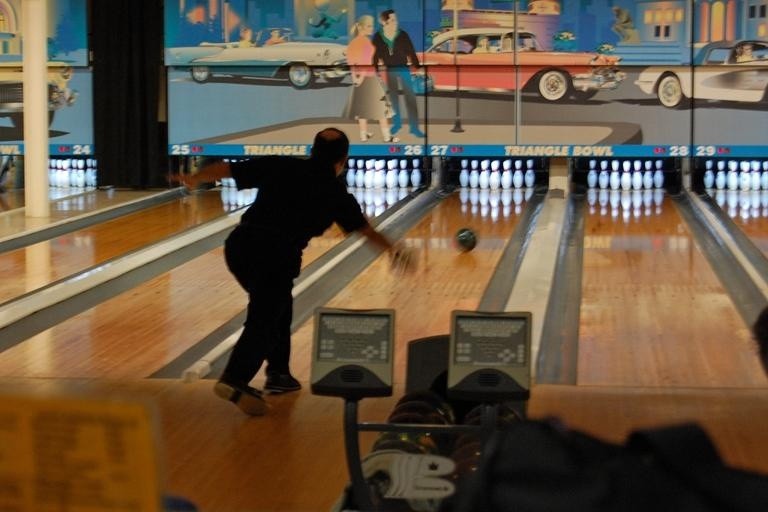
190;39;349;89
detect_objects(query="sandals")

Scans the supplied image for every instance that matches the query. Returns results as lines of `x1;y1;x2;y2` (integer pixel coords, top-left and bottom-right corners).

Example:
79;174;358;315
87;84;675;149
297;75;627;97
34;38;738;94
360;133;373;141
385;137;398;142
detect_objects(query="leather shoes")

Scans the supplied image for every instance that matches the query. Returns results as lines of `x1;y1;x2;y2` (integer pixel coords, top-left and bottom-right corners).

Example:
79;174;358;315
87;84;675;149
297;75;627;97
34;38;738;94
390;124;402;134
213;381;268;417
262;376;303;391
409;128;425;137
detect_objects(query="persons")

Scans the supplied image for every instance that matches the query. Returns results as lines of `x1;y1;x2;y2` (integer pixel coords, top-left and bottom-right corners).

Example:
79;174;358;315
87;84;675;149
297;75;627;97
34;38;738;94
469;32;539;55
369;9;427;142
264;29;286;46
166;127;407;421
737;41;768;64
340;12;400;143
237;26;255;48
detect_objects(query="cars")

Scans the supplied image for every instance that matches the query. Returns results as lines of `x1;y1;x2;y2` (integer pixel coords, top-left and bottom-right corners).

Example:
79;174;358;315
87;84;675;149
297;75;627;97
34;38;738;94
634;39;767;109
380;27;627;104
0;0;75;132
